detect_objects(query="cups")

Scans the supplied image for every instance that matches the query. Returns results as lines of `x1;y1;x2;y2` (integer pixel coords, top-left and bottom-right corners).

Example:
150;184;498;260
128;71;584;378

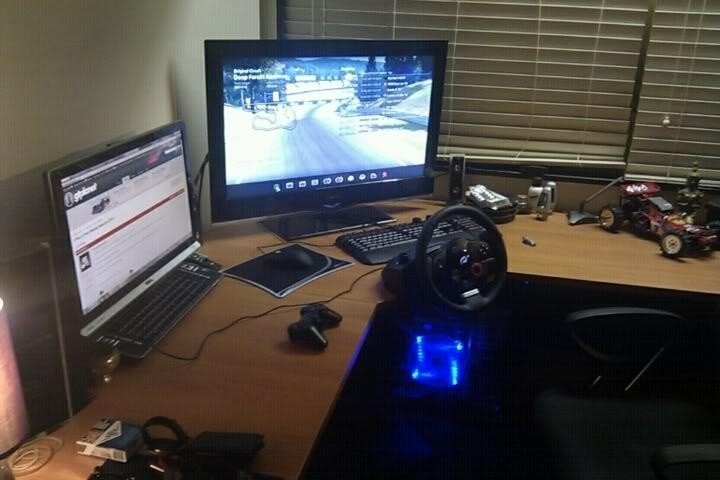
0;297;29;458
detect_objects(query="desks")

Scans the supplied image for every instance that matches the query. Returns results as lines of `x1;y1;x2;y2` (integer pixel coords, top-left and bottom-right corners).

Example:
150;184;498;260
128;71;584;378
193;197;454;307
0;248;381;480
417;189;720;300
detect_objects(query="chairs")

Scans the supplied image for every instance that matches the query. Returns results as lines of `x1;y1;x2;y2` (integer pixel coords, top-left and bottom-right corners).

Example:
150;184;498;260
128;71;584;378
530;298;719;480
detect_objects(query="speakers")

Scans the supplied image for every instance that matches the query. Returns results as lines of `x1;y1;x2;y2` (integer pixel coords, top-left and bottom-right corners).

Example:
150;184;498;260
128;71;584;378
447;154;466;205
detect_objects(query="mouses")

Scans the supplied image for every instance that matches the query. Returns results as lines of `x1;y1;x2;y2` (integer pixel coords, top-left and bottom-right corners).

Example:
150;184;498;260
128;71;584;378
271;246;314;268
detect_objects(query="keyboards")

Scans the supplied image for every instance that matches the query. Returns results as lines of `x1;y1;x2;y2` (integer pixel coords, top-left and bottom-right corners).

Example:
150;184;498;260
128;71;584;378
89;261;224;360
337;215;499;267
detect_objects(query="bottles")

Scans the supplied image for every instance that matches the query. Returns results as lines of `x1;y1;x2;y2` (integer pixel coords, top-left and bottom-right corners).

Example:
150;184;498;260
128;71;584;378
528;176;559;222
675;161;706;214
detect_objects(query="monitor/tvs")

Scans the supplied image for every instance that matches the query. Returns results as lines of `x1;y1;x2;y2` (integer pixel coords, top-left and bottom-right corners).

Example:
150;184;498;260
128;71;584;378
202;38;451;243
45;119;202;337
